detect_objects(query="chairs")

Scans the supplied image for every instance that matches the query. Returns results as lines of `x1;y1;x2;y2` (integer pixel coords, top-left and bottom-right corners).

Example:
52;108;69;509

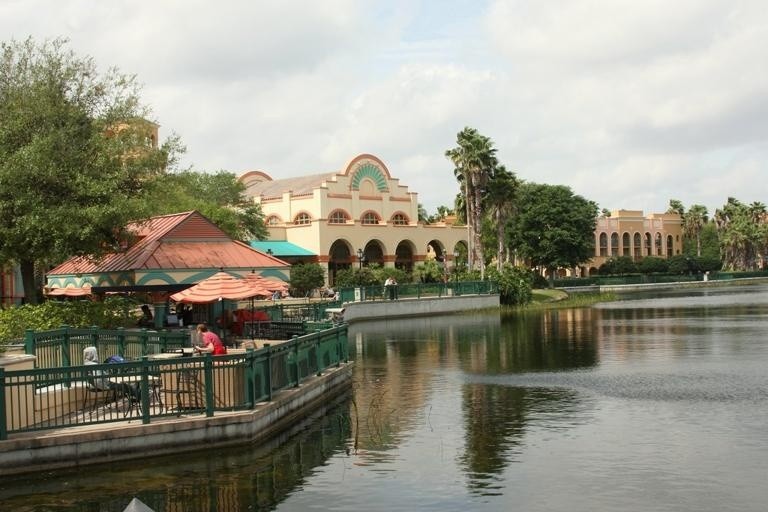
80;362;199;418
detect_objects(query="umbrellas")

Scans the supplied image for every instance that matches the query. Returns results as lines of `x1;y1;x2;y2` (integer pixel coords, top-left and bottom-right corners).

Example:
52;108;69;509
170;272;290;355
48;288;92;296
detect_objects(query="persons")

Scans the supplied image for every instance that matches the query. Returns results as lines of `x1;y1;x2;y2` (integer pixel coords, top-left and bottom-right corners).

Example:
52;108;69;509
83;346;153;400
383;277;398;300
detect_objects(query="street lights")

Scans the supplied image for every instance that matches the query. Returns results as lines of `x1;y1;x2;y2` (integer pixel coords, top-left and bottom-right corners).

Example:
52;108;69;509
454;248;459;266
609;258;613;278
357;249;363;287
686;256;690;277
